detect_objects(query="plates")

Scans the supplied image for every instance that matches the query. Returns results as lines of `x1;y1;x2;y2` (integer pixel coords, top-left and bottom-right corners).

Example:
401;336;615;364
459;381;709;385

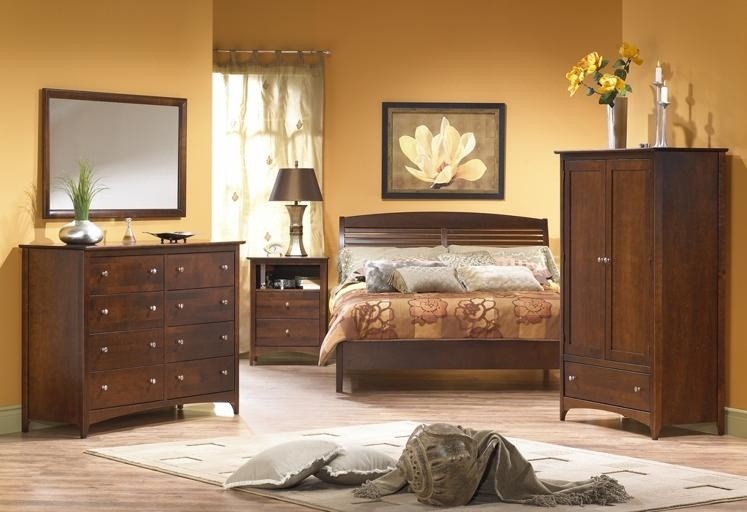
141;230;198;244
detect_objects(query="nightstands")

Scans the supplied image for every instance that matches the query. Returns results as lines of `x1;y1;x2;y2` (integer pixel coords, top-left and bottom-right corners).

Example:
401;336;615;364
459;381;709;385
245;255;327;366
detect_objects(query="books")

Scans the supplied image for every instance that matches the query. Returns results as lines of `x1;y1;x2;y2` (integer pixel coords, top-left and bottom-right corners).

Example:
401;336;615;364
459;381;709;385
295;276;320;289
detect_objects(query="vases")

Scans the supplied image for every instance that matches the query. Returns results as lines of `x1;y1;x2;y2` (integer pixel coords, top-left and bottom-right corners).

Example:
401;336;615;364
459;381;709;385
606;97;628;150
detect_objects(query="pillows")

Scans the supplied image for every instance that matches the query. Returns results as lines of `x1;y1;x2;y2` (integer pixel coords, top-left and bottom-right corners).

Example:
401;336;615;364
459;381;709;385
334;241;554;294
324;440;402;484
224;433;340;494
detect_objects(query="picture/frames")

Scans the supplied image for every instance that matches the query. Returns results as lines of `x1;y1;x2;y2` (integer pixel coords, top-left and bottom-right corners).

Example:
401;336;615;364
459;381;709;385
378;100;508;201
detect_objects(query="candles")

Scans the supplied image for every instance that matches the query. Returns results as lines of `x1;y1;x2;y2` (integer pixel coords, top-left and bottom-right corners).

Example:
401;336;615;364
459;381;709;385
659;81;667;103
654;60;661;84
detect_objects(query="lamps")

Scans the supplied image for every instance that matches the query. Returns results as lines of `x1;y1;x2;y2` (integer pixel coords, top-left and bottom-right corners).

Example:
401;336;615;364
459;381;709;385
266;157;324;256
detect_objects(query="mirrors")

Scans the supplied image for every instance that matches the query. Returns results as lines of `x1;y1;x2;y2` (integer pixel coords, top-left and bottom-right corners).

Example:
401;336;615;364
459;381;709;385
40;86;188;220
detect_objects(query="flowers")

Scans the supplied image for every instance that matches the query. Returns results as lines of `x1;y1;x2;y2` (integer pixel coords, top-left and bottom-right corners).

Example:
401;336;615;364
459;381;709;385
564;42;643;107
397;117;487;190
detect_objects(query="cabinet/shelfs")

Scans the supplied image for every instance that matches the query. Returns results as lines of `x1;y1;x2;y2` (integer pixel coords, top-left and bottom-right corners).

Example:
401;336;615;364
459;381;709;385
551;146;732;440
15;237;248;440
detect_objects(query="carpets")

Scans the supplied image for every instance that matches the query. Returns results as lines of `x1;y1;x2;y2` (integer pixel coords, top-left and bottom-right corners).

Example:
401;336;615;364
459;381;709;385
79;416;747;511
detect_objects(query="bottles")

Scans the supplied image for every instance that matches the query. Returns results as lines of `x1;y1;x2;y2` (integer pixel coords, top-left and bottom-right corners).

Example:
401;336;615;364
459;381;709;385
122;216;137;245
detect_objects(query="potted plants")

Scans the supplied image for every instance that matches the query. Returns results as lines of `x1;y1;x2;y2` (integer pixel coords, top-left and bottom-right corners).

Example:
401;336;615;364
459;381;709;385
51;155;113;247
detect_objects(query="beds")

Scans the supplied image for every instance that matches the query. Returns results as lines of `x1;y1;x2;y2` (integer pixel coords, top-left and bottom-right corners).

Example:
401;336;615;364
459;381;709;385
319;208;562;397
